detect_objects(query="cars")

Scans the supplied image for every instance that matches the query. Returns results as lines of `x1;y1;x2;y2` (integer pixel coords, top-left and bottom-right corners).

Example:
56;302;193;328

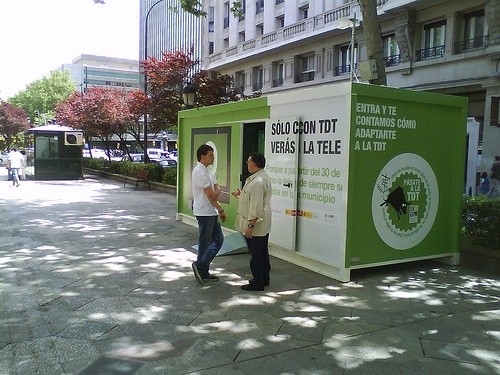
121;154;177;169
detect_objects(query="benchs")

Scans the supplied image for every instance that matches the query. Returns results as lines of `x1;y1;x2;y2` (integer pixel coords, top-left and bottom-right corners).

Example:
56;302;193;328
124;168;151;191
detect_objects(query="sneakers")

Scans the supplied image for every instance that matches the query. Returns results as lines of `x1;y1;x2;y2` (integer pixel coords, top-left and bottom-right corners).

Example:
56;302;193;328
203;273;218;281
192;262;204;285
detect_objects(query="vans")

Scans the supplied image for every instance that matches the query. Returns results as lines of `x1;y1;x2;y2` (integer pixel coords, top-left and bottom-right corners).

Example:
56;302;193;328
147;147;170;159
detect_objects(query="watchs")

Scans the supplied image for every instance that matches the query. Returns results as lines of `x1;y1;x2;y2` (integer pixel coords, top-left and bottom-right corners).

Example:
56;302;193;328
248;224;254;228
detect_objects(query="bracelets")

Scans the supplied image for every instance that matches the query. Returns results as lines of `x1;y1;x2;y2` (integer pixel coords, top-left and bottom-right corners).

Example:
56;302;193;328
219;209;224;213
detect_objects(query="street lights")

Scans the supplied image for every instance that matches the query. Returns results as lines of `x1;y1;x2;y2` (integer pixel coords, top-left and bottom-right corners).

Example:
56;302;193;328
83;63;88;92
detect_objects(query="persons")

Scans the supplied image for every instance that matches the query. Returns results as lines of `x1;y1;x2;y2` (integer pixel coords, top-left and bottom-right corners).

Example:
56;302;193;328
105;148;118;157
231;152;272;291
7;147;27;186
192;144;227;286
477;156;500;199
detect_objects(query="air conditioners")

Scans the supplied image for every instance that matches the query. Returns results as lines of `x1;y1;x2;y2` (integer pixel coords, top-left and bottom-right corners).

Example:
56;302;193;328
64;132;83;146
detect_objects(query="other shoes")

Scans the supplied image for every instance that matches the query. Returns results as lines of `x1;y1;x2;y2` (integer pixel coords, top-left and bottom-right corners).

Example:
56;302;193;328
241;285;257;290
249;279;257;284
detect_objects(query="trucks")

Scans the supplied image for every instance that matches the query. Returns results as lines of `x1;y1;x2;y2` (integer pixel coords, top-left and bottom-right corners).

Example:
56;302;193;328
83;149;109;162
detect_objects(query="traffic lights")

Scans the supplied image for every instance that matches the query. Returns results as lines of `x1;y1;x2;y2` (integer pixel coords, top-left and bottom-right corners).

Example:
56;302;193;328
35;111;39;117
